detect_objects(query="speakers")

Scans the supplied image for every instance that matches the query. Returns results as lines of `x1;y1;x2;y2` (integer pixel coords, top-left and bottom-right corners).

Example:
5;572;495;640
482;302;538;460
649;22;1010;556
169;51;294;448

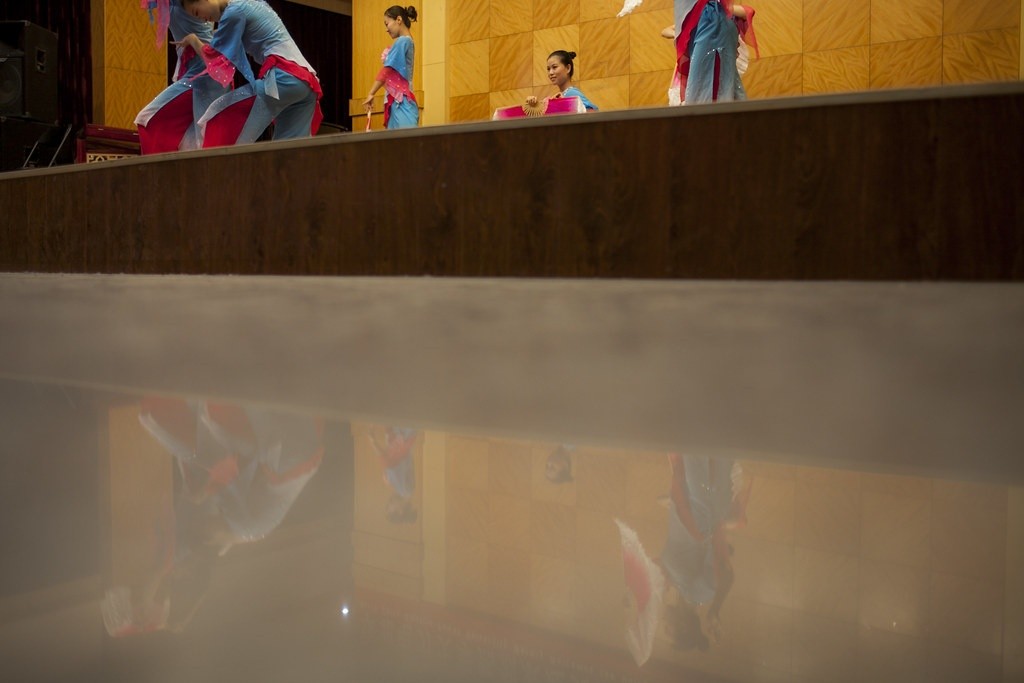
0;20;58;122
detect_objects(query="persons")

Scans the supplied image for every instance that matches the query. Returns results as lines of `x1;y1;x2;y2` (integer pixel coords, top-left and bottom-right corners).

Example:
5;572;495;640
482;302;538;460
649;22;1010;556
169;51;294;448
622;450;740;652
662;0;756;106
368;424;420;526
361;5;420;128
526;50;600;114
544;443;585;484
136;395;329;630
133;0;323;156
